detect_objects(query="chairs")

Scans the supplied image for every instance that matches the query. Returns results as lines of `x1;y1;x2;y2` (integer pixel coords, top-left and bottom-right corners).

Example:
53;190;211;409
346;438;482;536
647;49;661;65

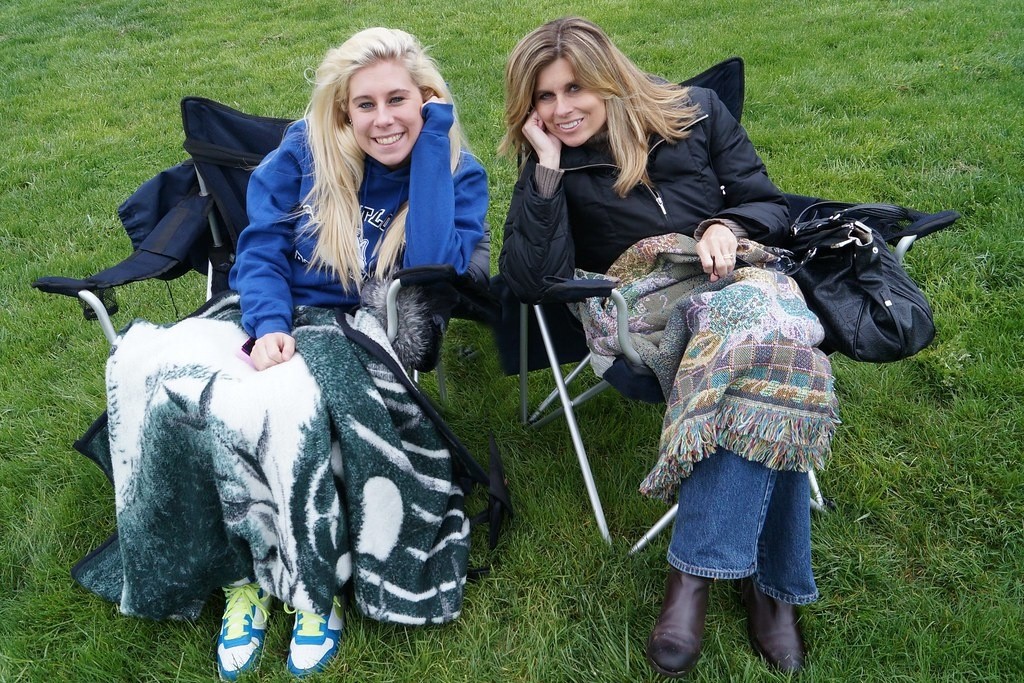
32;92;448;409
510;54;961;559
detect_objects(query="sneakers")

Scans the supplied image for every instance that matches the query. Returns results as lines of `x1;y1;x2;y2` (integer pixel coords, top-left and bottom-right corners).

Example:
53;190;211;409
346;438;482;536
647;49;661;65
217;580;271;683
284;595;347;679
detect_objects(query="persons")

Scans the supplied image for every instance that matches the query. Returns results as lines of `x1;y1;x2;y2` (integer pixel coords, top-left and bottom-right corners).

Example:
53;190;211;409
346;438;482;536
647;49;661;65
217;26;490;682
497;16;839;679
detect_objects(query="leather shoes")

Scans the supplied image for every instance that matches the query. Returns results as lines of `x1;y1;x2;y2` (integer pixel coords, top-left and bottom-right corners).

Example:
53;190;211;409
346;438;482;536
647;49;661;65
747;582;808;677
646;566;712;679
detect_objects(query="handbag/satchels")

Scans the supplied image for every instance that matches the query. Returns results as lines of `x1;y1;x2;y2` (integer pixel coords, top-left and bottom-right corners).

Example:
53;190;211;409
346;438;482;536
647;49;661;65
778;201;936;364
